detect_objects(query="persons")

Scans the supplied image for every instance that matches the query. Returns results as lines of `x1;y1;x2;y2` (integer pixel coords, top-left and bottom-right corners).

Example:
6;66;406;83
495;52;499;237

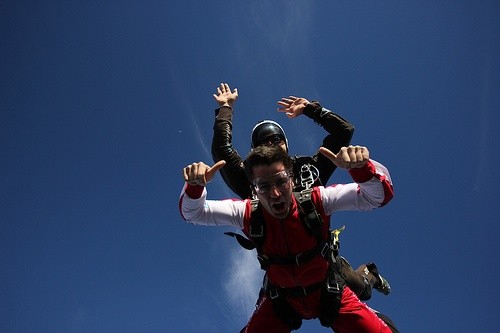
178;145;401;333
211;83;391;308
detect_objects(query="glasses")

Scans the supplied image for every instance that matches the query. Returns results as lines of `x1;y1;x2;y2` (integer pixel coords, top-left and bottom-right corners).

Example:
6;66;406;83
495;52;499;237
247;169;291;194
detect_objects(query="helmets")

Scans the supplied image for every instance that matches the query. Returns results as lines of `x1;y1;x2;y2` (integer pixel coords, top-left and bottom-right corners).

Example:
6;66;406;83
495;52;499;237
250;119;289;151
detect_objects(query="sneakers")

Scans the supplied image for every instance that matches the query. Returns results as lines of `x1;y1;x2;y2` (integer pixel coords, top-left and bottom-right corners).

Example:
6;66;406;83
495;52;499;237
376;274;392;295
